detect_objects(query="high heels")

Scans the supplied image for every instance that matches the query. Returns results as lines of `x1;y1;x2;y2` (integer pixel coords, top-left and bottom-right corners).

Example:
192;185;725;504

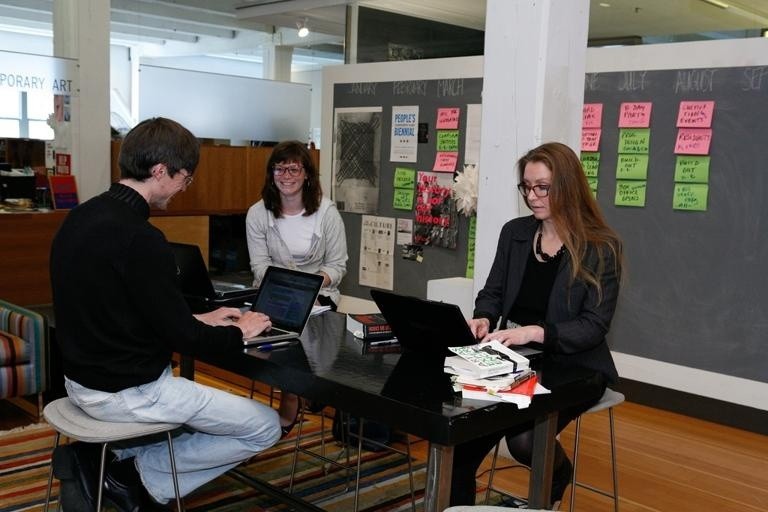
278;398;303;439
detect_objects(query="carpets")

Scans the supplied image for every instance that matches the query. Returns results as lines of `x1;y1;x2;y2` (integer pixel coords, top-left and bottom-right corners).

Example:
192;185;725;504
1;366;542;512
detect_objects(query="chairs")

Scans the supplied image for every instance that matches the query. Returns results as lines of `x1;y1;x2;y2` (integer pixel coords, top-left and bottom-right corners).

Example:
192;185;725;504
0;302;45;422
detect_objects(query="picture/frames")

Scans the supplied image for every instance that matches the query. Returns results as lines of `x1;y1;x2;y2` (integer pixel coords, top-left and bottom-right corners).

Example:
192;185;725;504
48;174;79;210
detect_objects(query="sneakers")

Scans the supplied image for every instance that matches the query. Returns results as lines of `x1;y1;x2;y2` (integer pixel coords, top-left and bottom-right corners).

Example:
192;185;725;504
551;457;572;503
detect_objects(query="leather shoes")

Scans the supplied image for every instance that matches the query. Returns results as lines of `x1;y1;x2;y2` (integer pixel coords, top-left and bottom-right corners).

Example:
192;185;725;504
52;445;98;512
91;468;144;512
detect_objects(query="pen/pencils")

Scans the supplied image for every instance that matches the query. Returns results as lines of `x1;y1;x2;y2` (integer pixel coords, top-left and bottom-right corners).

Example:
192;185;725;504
256;341;292;349
370;339;399;345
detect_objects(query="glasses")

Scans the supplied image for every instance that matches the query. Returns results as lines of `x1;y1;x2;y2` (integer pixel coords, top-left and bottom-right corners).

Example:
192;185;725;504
273;164;304;177
517;182;552;197
164;168;194;187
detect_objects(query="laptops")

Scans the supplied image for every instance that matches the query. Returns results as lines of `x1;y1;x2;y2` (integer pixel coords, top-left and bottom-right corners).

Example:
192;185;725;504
170;241;260;307
371;286;545;384
243;264;325;344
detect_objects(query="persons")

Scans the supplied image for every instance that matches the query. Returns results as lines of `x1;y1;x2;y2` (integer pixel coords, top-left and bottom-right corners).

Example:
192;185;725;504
448;141;622;512
245;139;350;439
51;115;281;511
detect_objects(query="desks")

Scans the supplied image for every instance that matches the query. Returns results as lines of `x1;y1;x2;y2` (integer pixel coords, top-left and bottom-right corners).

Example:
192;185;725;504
175;267;558;512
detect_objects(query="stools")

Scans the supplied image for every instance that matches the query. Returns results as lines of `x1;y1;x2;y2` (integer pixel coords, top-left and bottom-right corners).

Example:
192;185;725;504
41;399;184;512
288;397;416;512
249;378;304;417
484;385;625;512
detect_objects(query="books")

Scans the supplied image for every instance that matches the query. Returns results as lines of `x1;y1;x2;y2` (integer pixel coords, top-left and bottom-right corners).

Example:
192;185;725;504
443;340;530;380
461;375;540;404
449;369;529;386
347;312;393;340
453;371;536;392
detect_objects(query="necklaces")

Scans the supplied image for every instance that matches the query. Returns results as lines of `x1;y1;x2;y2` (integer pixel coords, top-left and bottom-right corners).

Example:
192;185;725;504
534;228;566;262
